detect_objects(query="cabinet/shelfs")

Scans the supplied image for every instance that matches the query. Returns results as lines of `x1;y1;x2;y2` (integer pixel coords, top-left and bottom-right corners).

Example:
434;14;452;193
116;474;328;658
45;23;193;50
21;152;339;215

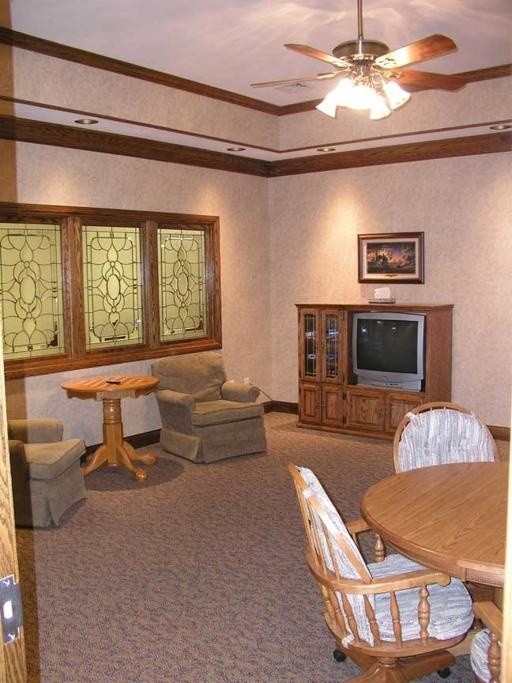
296;302;455;441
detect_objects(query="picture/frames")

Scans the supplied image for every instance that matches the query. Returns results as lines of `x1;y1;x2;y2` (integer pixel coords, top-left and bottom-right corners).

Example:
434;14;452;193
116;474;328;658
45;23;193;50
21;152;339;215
357;232;423;283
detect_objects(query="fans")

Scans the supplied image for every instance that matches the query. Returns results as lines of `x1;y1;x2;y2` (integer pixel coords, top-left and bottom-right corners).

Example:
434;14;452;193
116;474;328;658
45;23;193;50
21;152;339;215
248;0;468;91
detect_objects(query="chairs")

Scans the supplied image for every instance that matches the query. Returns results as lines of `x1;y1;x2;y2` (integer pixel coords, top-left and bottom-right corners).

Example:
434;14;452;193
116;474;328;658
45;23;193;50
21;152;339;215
9;418;86;529
393;401;501;473
151;351;267;464
287;463;512;682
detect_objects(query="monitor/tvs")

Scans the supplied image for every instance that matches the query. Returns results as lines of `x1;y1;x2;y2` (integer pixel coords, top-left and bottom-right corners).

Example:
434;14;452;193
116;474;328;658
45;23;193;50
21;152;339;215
351;312;425;392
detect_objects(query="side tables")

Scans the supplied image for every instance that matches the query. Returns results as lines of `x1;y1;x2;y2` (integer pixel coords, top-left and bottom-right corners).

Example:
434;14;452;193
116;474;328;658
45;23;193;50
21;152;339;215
61;374;160;484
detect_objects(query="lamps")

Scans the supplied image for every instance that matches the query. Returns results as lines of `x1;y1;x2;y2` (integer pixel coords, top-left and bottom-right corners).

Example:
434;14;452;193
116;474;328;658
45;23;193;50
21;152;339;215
315;63;410;119
